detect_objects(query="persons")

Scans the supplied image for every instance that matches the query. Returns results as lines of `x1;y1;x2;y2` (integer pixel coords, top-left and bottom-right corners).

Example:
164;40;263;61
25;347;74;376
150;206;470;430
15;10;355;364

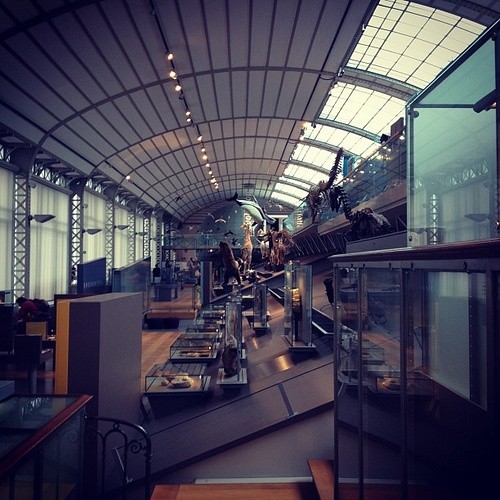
153;263;161;280
15;297;50;326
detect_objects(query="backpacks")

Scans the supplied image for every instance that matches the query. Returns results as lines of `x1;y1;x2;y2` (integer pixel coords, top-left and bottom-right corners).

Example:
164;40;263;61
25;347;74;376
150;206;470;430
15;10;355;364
27;299;50;312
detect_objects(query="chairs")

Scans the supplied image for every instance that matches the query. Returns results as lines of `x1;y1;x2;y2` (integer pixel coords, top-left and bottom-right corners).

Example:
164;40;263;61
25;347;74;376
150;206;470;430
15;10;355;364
15;334;53;394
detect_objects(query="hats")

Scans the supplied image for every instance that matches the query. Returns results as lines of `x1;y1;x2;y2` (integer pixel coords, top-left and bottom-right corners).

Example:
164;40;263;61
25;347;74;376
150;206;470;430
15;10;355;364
224;231;233;236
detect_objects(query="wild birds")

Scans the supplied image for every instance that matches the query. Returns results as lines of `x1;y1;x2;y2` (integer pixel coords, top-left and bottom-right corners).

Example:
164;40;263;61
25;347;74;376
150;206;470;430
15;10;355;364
215;216;226;223
223;230;237;236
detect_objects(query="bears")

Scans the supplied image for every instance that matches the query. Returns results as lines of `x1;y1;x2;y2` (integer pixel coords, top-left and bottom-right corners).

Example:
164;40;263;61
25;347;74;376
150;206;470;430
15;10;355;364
208;241;242;287
